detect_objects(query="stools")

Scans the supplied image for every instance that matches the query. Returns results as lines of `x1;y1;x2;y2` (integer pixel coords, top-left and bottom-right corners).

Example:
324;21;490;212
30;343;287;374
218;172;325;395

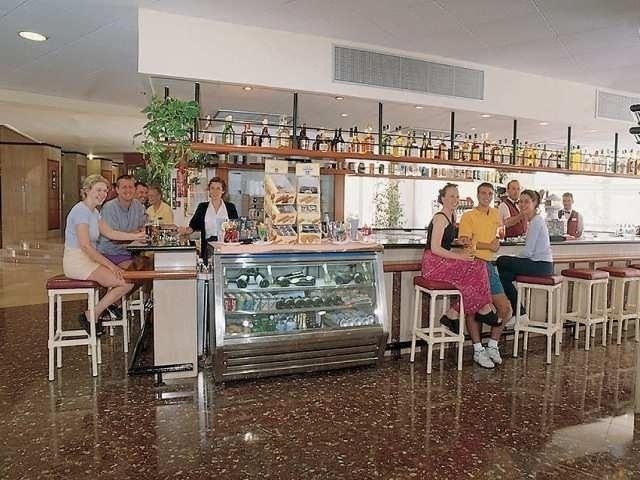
88;284;130;357
561;269;609;350
512;274;561;364
599;266;639;343
125;285;144;332
410;276;465;374
46;274;103;380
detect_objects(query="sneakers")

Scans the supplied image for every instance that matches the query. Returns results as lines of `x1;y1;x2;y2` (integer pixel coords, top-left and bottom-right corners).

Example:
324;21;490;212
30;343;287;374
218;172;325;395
486;346;502;364
473;348;495;369
79;304;121;337
504;313;528;327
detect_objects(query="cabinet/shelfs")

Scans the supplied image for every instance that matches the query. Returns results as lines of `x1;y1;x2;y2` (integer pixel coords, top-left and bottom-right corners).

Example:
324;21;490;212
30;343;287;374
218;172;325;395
154;82;640;184
206;240;390;383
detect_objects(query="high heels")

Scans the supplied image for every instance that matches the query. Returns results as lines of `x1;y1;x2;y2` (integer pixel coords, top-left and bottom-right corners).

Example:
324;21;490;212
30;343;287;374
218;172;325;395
440;315;459;333
475;311;502;326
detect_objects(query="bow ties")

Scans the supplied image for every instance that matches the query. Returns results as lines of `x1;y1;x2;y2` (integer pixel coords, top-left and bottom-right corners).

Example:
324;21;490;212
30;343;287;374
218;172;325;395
563;210;570;214
514;201;519;204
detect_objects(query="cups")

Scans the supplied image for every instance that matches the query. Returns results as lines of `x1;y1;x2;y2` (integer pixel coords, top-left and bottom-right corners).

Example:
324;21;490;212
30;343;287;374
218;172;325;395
144;214;191;245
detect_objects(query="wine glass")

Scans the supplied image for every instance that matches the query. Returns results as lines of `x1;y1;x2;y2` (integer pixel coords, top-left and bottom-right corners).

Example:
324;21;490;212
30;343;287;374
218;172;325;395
257;225;268;245
498;225;506;241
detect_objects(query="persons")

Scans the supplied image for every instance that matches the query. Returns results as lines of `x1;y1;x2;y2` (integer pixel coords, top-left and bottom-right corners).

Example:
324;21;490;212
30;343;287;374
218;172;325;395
499;179;527;237
558;193;583;241
177;176;238;266
146;186;174;225
496;189;553;327
95;175;159;320
63;174;156;337
422;185;502;335
134;182;149;204
457;182;510;368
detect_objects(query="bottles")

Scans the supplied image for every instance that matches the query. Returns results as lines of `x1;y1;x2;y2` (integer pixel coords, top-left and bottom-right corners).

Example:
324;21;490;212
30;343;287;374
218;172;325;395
322;211;330;233
199;113;640;184
236;262;375;332
